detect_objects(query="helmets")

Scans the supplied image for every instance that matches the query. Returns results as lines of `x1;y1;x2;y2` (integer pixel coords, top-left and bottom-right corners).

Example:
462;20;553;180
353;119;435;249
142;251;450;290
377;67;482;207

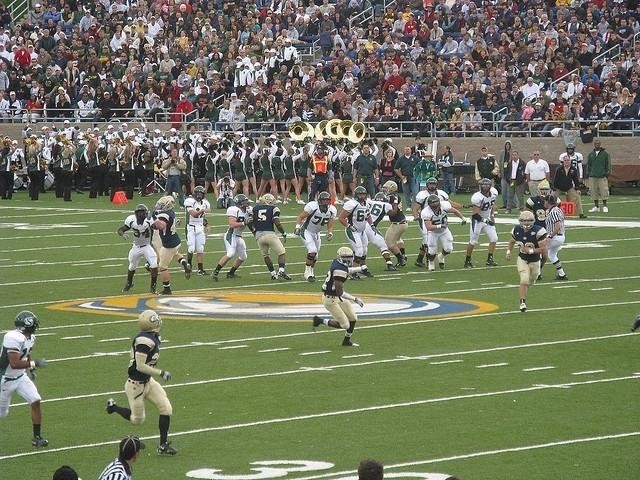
14;311;39;333
233;193;274;206
354;187;367;200
538;182;549;197
427;178;438;207
135;196;175;218
479;179;491;191
519;211;534;229
567;143;574;149
374;181;398;201
336;246;354;268
137;309;162;333
317;192;330;206
192;186;206;199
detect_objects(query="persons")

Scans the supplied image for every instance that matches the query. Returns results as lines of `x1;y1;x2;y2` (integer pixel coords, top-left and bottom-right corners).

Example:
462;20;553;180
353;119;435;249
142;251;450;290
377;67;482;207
184;186;210;274
52;465;78;480
294;192;337;282
248;194;292;280
1;0;640;136
421;195;466;270
212;194;253;281
544;195;567;280
413;177;470;267
97;433;146;480
0;120;611;217
506;211;548;312
358;457;384;480
526;183;561;229
117;197;191;296
0;309;51;447
105;309;180;454
465;178;498;268
339;181;408;279
312;247;367;347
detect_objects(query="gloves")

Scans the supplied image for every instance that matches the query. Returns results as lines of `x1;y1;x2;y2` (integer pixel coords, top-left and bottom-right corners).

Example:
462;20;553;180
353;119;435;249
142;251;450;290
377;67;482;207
294;228;301;235
160;370;171;381
461;220;466;226
463;205;474;208
30;358;46;370
437;224;446;229
29;372;37;380
356;299;364;307
282;232;287;243
327;234;333;241
122;234;129;239
371;225;383;236
485;216;495;225
349;224;357;232
243;217;253;225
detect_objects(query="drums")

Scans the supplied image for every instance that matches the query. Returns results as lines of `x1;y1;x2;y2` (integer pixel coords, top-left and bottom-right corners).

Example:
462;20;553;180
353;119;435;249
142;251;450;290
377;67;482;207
44;170;55;190
13;167;31;190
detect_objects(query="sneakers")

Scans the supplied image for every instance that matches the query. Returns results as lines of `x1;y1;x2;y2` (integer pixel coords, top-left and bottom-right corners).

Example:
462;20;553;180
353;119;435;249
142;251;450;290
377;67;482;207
603;206;608;212
342;341;359;347
157;443;178;455
386;256;407;271
464;263;473;269
118;436;146;457
589;207;600;212
505;209;512;214
350;269;373;281
555;274;568;281
334;197;351;205
33;438;48;447
313;316;319;332
499;206;506;209
151;282;172;295
415;255;445;271
227;274;240;278
304;272;316;282
538;275;542;280
276;196;305;204
520;303;527;312
183;263;218;280
486;260;497;266
271;272;291;280
406;207;412;212
581;215;587;219
145;262;150;272
106;398;115;415
122;283;134;292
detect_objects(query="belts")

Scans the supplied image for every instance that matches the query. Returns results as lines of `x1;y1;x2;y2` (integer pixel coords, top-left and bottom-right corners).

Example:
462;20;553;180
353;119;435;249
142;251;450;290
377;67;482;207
236;235;242;237
392;220;406;225
140;245;146;248
190;225;195;227
557;234;563;236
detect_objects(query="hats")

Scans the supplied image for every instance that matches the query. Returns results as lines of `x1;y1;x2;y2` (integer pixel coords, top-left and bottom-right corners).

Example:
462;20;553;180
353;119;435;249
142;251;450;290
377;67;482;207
422;152;434;158
14;141;18;144
65;119;69;124
545;196;558;204
209;130;243;140
26;128;36;139
42;126;50;132
93;123;177;134
5;4;291;97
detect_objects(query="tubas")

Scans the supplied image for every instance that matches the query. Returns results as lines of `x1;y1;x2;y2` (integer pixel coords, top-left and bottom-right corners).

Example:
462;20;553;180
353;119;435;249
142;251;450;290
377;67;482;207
289;118;366;145
